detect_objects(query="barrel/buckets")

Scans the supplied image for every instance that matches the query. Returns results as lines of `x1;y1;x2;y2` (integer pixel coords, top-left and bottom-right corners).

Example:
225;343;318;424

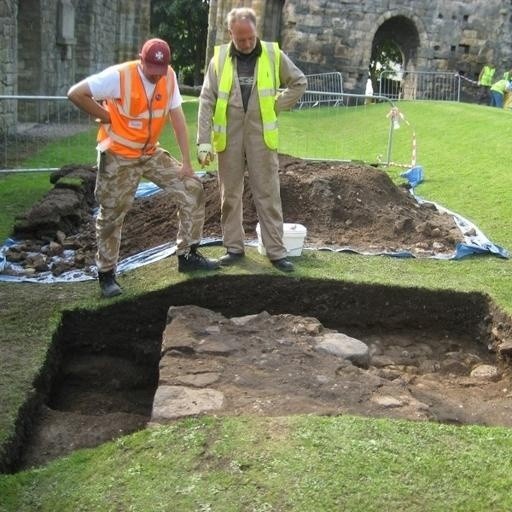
255;220;307;256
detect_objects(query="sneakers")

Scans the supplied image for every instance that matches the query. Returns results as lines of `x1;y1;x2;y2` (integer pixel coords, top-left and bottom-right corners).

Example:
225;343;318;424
178;242;220;272
98;269;123;297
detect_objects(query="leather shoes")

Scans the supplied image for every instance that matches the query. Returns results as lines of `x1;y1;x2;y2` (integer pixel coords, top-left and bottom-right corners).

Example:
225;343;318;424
221;251;245;265
271;259;295;272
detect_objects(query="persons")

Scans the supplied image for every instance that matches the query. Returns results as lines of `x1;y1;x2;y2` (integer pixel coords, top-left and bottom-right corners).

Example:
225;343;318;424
66;38;221;298
197;9;308;273
477;61;512;110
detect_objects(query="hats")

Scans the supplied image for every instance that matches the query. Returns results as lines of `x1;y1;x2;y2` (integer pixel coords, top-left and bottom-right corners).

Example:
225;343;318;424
140;38;171;77
